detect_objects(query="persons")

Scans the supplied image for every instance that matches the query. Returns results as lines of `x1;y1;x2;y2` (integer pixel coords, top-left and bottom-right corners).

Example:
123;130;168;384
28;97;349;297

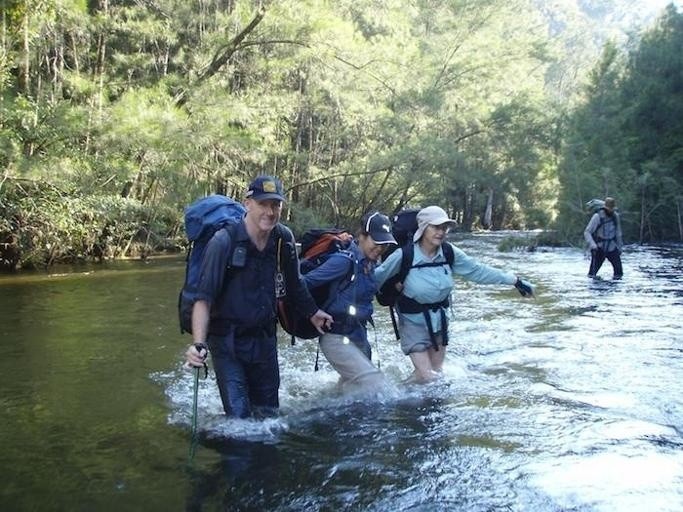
584;196;623;282
183;176;333;422
373;206;534;385
300;208;400;397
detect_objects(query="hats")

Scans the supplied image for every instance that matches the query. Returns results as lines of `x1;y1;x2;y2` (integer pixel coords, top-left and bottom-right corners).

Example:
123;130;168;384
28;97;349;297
413;205;458;243
603;197;619;211
361;209;398;245
246;175;288;205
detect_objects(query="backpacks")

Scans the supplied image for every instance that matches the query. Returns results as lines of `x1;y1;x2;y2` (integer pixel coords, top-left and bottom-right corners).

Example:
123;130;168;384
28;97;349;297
376;210;456;314
585;198;618;243
178;193;285;336
279;228;358;339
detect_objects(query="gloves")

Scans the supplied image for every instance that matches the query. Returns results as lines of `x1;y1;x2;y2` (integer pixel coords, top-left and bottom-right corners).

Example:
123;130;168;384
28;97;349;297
514;277;533;299
